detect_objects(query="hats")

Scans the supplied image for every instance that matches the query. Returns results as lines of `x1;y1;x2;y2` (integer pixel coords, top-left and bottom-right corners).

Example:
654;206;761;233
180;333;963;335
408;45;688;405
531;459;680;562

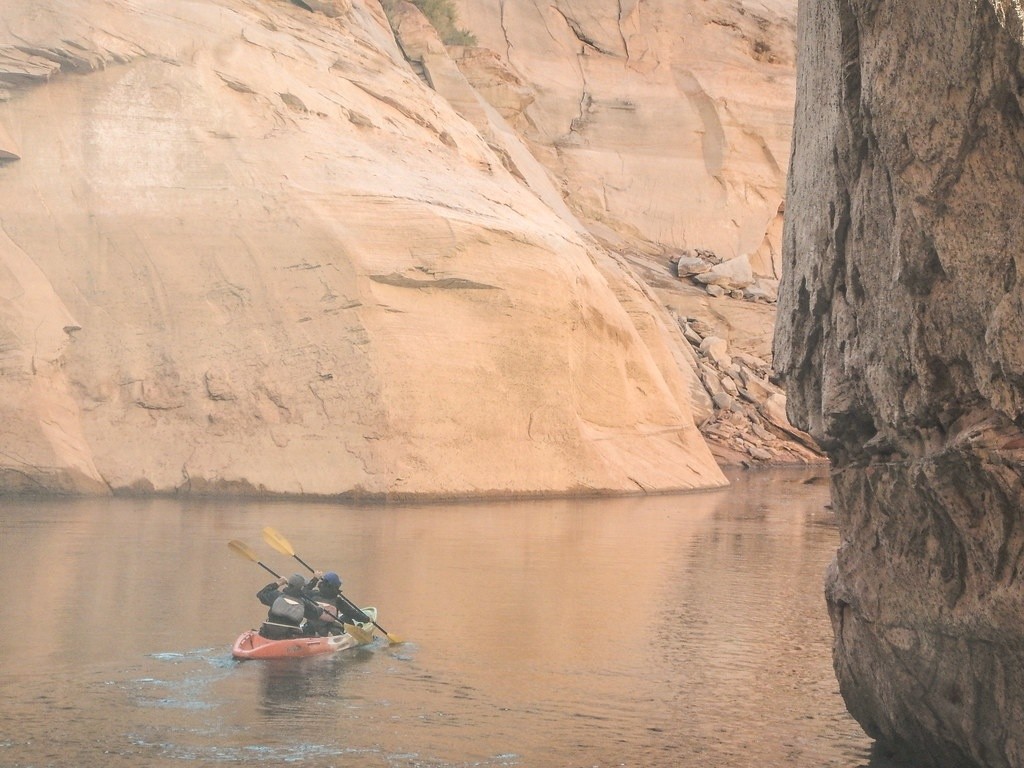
288;574;307;586
323;572;343;585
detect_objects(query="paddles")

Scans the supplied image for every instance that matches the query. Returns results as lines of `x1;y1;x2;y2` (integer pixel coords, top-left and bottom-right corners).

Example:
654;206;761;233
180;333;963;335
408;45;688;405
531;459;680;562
227;538;374;645
258;526;400;644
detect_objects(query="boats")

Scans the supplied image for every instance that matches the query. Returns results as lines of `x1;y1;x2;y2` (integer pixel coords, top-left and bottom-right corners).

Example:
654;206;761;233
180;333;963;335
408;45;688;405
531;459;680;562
233;606;378;660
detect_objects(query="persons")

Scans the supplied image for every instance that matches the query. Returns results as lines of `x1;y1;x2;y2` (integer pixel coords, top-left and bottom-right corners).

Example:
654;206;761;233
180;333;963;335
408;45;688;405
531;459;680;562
256;572;373;640
